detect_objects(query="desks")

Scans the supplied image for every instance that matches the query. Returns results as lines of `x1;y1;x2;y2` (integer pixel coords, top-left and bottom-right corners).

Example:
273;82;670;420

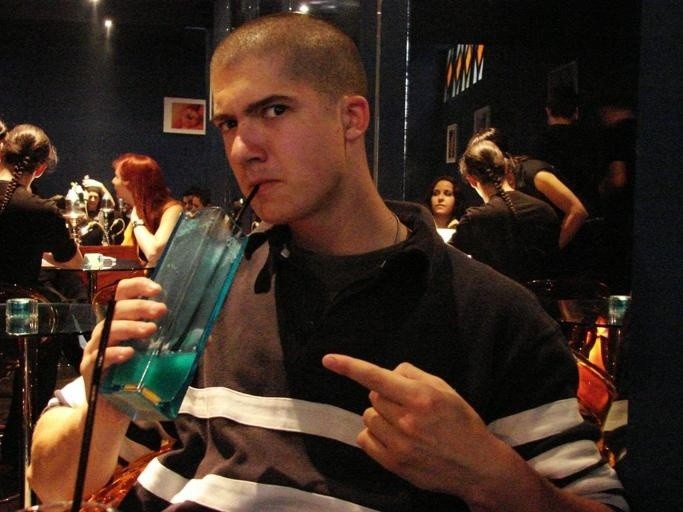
0;254;160;508
536;297;623;376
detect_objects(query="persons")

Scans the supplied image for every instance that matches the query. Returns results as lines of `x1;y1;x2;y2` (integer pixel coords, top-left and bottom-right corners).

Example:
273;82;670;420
420;81;635;298
28;13;631;512
1;121;229;473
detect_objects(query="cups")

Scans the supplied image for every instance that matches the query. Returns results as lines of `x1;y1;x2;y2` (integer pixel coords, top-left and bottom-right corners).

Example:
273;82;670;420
98;205;250;423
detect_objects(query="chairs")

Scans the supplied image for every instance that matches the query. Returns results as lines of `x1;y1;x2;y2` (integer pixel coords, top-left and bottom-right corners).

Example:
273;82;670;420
523;276;610;355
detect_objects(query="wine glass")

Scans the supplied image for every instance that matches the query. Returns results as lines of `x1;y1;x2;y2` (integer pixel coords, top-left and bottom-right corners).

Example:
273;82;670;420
63;214;85;243
98;208;115;245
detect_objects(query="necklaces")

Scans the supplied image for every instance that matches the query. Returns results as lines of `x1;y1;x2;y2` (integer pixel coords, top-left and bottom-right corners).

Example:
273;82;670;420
390;211;401;244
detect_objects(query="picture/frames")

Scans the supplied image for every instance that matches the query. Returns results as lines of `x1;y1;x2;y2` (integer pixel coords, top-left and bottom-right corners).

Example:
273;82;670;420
473;105;492;132
446;123;458;165
163;96;207;136
546;60;579;120
